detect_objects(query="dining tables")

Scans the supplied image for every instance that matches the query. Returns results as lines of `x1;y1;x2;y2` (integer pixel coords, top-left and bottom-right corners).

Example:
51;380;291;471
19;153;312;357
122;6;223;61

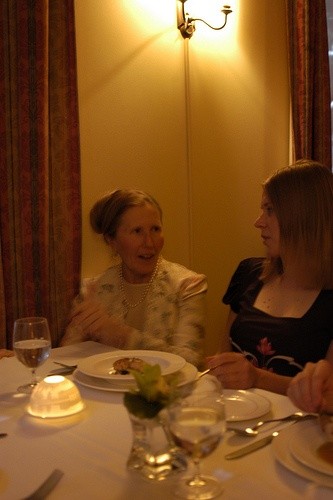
0;340;333;500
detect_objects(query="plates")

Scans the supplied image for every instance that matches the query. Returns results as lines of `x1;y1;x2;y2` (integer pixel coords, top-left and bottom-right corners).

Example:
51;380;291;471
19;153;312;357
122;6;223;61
222;388;273;421
287;415;333;476
271;427;333;488
73;362;198;392
77;349;187;381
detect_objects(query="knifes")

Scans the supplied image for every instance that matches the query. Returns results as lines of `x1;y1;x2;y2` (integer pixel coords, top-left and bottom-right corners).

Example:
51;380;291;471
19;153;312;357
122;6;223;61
223;413;318;460
47;365;77;376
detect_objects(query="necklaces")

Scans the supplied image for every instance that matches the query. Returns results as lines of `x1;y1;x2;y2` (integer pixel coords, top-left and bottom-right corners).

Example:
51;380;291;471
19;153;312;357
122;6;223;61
119;259;160;309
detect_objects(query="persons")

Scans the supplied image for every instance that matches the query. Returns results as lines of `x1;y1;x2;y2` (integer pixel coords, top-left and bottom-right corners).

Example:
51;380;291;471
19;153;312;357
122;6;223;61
201;159;333;415
58;188;208;368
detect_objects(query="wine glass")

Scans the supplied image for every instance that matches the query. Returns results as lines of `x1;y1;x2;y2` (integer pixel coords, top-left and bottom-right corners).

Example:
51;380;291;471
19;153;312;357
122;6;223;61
160;392;228;500
13;317;52;394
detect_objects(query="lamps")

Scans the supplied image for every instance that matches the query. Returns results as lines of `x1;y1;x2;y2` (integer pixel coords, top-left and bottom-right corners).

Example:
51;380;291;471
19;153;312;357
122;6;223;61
177;0;239;39
